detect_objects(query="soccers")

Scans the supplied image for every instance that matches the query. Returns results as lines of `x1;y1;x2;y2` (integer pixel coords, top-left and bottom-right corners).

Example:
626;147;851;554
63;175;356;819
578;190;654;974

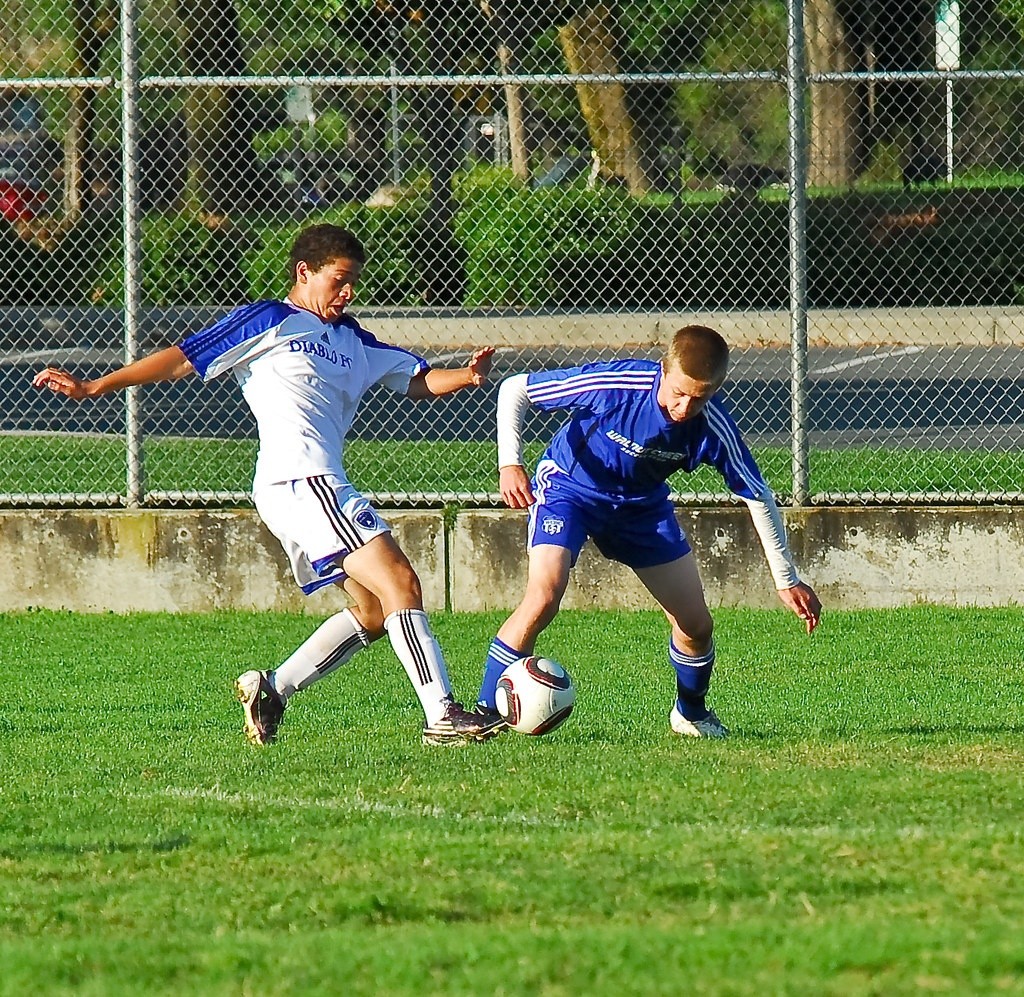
495;654;574;736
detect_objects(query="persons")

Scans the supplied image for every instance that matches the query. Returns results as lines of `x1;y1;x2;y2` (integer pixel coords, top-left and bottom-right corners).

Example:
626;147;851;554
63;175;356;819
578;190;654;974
472;324;821;736
32;224;508;747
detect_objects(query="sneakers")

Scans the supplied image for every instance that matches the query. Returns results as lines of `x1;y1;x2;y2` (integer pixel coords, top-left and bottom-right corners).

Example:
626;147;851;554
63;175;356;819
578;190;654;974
236;668;289;745
669;699;726;737
422;702;507;746
473;703;510;732
